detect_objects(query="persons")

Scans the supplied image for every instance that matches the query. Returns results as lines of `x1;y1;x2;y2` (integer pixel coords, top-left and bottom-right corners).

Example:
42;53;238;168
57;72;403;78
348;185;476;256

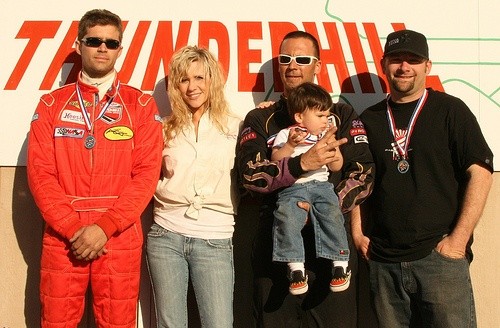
271;83;351;295
231;31;375;327
27;10;164;328
144;46;274;328
350;31;494;328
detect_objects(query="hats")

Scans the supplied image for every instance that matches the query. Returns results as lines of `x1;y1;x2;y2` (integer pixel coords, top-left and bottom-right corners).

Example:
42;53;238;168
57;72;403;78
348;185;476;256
384;30;429;60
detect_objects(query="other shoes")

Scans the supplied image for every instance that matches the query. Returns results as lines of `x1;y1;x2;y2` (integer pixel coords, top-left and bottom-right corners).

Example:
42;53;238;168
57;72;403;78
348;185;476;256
329;266;352;292
289;268;308;295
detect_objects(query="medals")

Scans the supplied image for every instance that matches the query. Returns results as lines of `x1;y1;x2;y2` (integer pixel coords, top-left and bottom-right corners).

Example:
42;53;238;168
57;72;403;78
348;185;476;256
85;136;95;148
398;160;409;174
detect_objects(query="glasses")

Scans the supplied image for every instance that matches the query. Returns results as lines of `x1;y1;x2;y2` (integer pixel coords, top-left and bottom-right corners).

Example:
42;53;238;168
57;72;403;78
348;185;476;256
278;55;318;65
80;37;121;49
